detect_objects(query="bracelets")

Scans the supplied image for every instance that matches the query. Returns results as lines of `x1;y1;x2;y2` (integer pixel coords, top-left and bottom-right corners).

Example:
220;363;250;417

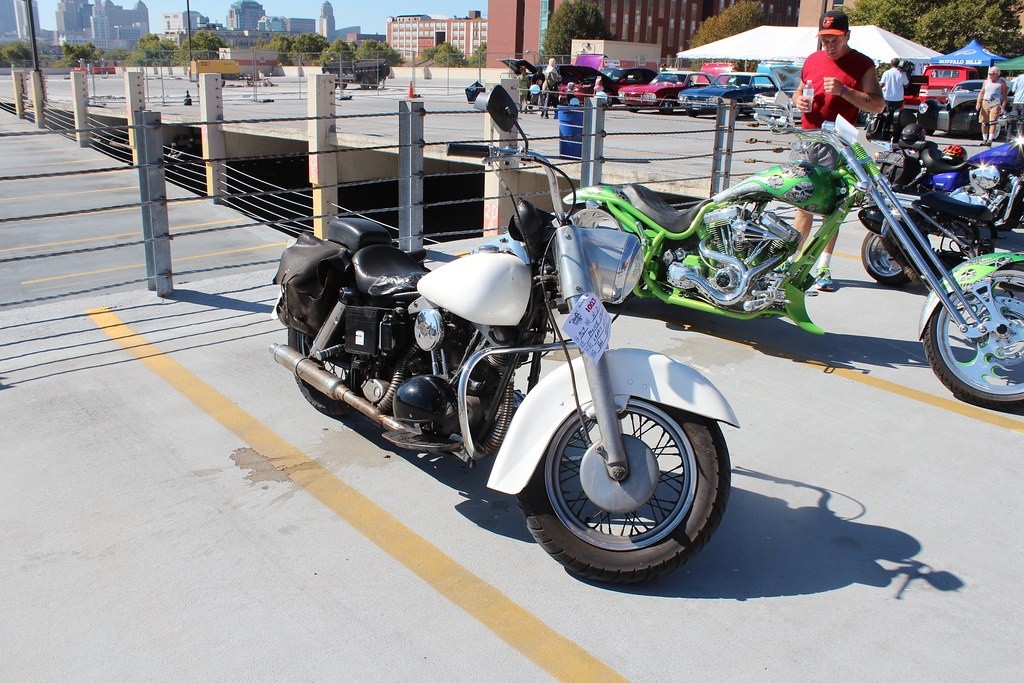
840;85;847;96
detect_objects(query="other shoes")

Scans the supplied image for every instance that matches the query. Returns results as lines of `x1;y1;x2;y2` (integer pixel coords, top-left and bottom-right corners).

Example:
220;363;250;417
986;140;992;145
980;141;986;146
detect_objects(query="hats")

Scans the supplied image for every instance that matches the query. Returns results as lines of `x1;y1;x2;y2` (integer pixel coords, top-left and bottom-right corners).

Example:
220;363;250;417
817;10;849;35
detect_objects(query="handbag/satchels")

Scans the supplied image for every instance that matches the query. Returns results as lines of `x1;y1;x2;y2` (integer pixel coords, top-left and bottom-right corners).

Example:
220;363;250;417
550;84;558;92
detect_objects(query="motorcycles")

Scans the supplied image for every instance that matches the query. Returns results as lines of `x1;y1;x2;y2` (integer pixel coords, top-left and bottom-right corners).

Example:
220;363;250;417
267;83;741;585
857;117;1024;287
562;112;1024;415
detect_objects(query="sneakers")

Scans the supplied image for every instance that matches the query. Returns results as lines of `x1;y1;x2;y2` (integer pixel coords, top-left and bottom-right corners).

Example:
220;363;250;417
773;261;795;272
816;266;837;291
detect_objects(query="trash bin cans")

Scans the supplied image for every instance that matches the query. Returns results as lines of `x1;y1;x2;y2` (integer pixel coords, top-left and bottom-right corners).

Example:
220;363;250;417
558;105;584;159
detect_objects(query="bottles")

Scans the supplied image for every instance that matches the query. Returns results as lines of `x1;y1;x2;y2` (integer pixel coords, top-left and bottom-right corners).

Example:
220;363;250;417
802;80;814;112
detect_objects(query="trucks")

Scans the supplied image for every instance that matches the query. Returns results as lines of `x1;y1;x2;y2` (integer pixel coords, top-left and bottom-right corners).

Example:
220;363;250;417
321;59;391;90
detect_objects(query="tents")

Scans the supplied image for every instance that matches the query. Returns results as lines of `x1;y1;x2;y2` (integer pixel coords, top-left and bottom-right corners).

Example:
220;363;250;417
995;55;1024;71
676;25;944;75
931;38;1008;70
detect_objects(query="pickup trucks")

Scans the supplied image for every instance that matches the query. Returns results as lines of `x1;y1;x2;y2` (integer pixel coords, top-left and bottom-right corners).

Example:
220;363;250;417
676;71;796;120
900;64;980;111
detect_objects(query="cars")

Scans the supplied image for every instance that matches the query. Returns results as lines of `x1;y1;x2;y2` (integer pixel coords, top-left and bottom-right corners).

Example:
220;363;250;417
617;71;712;115
915;79;983;140
497;54;670;106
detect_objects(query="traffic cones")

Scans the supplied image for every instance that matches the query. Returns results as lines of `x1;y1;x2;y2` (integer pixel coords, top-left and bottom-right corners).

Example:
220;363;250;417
408;81;414;98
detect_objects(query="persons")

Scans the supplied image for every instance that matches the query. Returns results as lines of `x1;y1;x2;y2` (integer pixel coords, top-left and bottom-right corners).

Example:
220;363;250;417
566;82;583;105
660;63;667;72
517;58;562;120
976;67;1007;147
593;78;604;94
1007;73;1024;142
786;10;885;292
880;58;909;145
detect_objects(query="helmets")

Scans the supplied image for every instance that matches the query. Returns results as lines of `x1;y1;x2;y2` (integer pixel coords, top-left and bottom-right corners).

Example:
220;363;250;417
942;144;969;163
901;123;925;141
